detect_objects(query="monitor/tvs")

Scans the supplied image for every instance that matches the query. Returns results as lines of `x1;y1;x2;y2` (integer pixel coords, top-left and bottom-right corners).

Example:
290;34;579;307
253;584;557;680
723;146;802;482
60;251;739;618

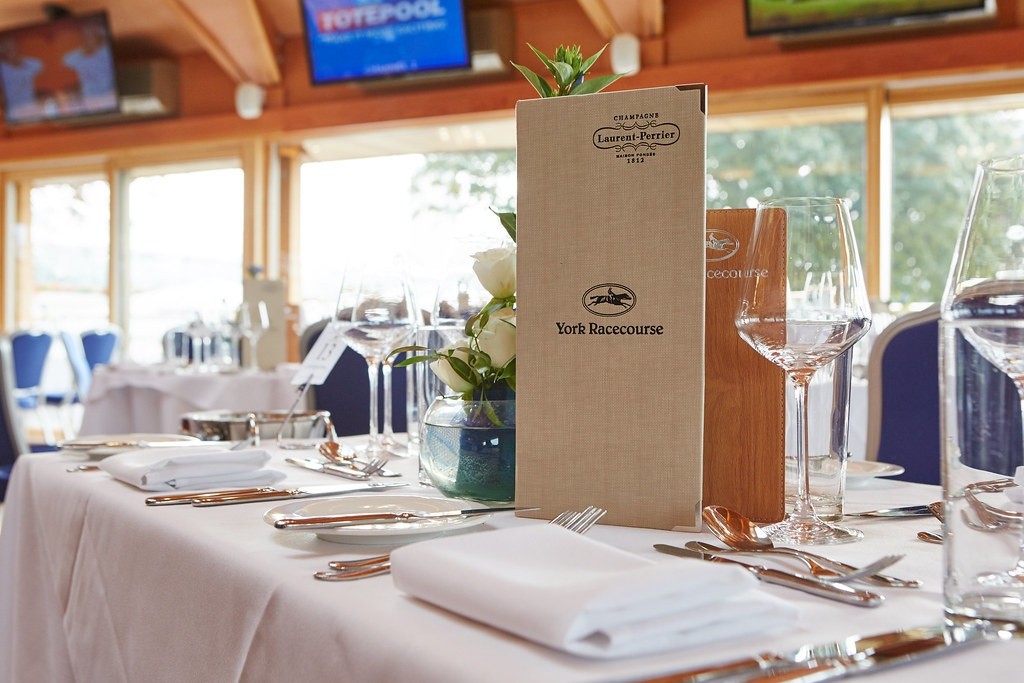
0;9;121;128
742;0;997;38
298;0;472;87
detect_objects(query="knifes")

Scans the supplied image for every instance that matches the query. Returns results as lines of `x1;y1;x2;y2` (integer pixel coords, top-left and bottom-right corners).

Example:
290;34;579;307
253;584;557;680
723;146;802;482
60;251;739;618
192;483;409;507
274;506;543;529
654;544;884;608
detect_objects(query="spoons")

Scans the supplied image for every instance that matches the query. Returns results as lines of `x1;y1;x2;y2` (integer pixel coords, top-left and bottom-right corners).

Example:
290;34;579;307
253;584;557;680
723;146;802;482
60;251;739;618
703;505;918;589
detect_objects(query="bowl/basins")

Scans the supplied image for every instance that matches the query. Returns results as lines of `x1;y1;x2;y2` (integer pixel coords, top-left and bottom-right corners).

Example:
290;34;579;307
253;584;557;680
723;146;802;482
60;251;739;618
181;411;330;443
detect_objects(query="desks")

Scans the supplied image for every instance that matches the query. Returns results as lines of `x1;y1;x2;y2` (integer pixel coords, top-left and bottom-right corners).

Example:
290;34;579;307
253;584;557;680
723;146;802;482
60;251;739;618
77;362;317;438
1;432;1024;682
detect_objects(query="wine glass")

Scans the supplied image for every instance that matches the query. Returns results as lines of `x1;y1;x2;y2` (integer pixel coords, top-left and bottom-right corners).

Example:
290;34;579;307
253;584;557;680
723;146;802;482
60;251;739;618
940;159;1023;515
735;196;873;546
330;268;495;464
238;300;270;373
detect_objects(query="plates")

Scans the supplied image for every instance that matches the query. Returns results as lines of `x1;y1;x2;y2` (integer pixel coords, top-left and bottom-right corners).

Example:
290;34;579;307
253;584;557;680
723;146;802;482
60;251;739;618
57;433;200;458
263;494;492;546
785;456;906;487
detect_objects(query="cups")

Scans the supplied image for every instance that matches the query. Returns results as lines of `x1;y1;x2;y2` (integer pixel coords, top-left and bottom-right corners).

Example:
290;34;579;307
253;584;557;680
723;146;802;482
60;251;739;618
783;309;852;524
938;321;1023;644
416;325;479;435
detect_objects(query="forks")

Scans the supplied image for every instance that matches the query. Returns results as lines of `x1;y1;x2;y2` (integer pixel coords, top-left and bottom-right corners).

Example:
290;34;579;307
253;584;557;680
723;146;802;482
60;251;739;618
685;541;906;583
314;504;608;583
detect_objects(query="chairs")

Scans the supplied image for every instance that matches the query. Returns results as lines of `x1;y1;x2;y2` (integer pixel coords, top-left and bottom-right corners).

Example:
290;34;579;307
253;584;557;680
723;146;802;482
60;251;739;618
0;278;1024;505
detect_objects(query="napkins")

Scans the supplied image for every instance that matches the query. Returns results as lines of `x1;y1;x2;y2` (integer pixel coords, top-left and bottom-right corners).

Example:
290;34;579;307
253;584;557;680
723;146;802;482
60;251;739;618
99;442;288;494
388;523;795;659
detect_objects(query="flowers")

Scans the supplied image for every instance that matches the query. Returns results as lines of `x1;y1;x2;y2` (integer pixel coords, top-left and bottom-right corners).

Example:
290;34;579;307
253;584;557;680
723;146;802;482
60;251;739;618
386;207;516;427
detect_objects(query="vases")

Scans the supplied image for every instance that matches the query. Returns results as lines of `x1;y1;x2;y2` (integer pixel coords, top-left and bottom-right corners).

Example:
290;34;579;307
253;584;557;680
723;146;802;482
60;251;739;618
420;395;517;503
